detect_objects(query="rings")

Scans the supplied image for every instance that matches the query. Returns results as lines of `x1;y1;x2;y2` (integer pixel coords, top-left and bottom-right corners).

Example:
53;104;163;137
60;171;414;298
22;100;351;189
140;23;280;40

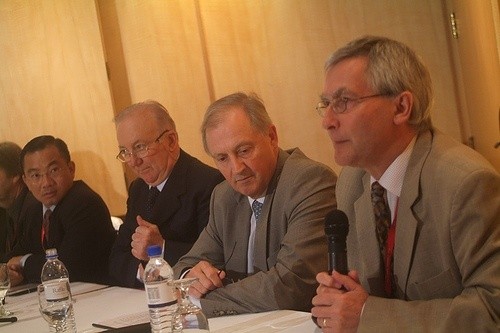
323;318;328;326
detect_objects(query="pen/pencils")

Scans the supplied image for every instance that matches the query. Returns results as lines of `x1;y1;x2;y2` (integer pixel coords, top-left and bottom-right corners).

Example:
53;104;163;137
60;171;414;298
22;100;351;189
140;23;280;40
92;324;115;329
9;287;38;296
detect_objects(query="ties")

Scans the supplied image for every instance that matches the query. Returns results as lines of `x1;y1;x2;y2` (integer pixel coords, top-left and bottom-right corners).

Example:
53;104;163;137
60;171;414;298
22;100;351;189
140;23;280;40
371;180;394;291
252;200;263;225
42;209;53;249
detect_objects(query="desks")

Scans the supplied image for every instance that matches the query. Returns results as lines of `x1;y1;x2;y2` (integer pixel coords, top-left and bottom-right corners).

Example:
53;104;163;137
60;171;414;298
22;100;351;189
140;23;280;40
0;274;324;333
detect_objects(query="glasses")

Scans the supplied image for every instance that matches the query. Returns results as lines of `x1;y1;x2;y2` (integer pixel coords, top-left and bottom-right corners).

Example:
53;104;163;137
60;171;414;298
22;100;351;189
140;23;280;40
316;91;389;118
116;129;170;164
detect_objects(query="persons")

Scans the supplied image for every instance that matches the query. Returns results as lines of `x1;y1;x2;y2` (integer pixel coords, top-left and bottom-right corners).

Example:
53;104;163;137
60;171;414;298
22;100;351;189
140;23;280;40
0;135;117;287
0;141;43;282
106;99;226;289
170;90;338;319
311;35;500;333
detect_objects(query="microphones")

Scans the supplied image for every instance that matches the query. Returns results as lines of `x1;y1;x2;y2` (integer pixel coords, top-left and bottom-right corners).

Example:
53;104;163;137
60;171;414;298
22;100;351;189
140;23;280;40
325;209;349;274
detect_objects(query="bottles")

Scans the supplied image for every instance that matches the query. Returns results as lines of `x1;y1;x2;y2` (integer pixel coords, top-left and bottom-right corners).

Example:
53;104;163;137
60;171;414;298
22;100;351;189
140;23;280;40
40;247;78;333
141;245;176;332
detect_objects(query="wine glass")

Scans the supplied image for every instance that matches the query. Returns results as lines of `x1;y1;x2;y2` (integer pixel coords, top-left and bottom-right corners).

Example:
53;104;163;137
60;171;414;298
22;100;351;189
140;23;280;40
175;277;210;332
38;280;73;333
0;263;15;317
